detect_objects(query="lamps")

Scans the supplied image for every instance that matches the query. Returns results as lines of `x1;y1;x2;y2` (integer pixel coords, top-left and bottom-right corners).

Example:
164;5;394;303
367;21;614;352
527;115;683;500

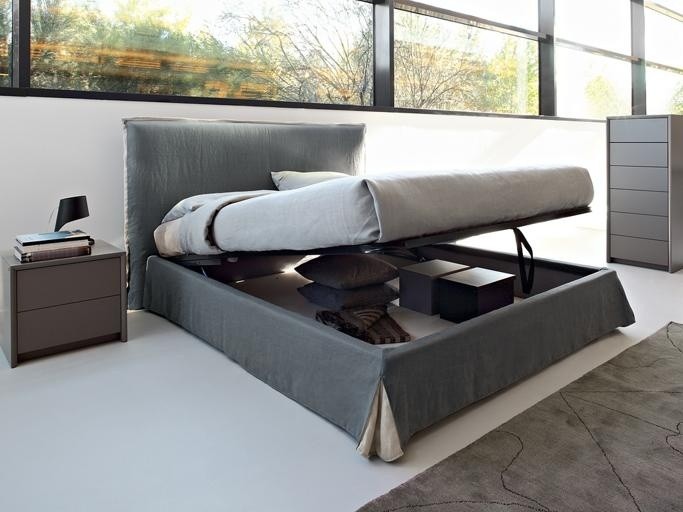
53;195;89;232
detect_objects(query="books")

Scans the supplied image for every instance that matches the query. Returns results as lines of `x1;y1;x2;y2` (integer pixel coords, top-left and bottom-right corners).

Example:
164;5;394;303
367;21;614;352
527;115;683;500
12;245;91;264
14;230;89;254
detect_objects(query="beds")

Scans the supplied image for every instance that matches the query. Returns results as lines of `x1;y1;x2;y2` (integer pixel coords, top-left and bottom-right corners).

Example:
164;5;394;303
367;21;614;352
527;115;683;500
120;118;635;464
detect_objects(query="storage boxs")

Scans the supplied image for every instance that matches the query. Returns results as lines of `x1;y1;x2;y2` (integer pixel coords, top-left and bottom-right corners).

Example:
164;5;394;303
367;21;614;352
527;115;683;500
398;259;516;326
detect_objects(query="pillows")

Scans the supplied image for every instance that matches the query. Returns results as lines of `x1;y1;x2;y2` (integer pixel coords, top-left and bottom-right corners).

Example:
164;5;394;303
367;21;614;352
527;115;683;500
294;253;400;314
161;171;352;228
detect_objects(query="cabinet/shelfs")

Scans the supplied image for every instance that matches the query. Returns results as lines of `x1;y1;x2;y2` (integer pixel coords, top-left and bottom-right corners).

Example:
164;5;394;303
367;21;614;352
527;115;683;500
606;114;683;273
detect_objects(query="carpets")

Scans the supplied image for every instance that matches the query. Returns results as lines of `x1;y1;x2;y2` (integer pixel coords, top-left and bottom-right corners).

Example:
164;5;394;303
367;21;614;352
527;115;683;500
353;321;683;512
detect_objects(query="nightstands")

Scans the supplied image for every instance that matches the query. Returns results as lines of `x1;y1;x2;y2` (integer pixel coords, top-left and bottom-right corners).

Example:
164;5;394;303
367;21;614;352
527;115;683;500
0;230;128;369
